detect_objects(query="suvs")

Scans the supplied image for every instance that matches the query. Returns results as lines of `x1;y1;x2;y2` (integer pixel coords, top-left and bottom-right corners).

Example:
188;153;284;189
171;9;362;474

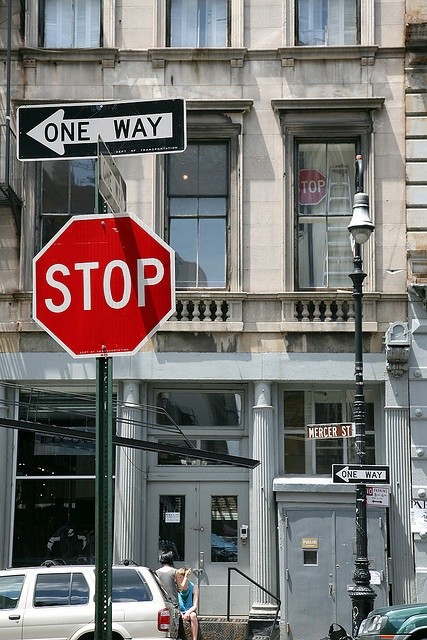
0;559;179;640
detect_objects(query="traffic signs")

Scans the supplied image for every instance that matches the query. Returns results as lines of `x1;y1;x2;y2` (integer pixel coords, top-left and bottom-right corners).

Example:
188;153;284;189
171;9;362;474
12;96;188;163
304;422;355;440
330;462;390;487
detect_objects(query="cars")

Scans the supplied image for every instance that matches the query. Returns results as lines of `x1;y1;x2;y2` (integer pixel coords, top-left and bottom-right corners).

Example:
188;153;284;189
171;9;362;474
357;603;426;639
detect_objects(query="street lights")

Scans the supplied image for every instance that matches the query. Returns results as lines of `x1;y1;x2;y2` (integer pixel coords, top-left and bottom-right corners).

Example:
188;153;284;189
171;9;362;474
345;157;376;638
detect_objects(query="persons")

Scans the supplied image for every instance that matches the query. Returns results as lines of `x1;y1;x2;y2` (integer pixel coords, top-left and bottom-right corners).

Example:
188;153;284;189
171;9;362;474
177;568;200;640
155;553;191;640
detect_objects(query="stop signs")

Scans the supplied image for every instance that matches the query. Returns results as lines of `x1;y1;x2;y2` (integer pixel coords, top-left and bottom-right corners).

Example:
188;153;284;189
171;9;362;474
297;169;327;204
31;212;177;361
